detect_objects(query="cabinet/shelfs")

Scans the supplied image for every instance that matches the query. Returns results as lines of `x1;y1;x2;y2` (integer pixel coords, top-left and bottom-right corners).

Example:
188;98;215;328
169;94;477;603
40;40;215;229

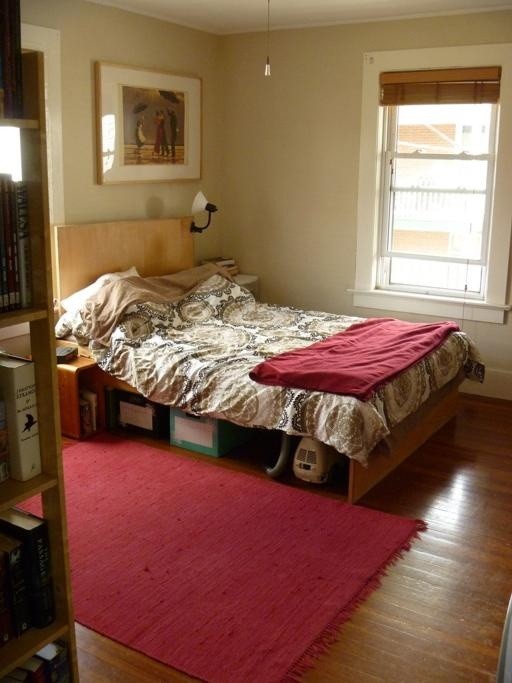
1;45;82;681
56;345;104;442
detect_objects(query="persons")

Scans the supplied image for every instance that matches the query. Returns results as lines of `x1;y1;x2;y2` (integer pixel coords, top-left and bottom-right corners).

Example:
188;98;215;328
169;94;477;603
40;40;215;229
136;112;146;147
154;108;178;156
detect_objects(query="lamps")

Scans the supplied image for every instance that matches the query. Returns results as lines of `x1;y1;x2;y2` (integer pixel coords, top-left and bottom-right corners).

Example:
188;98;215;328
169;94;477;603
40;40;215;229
187;190;217;233
263;1;272;78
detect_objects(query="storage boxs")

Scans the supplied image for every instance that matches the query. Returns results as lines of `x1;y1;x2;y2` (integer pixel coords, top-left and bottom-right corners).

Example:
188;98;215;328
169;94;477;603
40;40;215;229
167;407;241;459
113;395;168;440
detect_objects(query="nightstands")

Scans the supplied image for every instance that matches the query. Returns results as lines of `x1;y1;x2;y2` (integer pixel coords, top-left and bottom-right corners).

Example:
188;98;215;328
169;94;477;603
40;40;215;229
233;271;261;304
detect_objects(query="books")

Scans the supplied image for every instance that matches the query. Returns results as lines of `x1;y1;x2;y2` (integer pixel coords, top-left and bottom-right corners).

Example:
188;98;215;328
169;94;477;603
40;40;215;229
0;173;77;682
80;390;98;432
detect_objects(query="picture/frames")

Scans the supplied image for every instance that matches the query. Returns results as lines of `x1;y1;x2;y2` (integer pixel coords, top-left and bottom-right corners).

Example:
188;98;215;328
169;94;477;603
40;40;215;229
92;60;203;185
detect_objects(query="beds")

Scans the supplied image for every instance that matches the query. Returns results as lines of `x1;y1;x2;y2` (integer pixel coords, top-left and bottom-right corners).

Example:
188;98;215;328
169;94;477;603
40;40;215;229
49;213;489;509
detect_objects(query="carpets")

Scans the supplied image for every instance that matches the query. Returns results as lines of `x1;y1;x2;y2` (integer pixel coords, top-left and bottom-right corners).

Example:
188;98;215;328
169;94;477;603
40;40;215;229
19;430;427;678
